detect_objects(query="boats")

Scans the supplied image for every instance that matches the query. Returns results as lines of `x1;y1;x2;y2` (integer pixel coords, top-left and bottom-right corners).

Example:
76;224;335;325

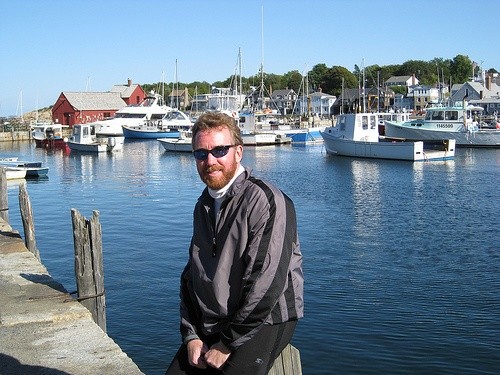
0;157;49;180
67;124;117;152
86;5;500;163
30;122;69;146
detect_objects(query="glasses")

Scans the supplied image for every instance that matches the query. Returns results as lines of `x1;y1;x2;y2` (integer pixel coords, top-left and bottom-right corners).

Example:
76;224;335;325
193;145;235;160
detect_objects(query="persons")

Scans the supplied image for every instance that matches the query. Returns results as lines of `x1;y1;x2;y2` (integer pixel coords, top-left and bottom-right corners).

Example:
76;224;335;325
472;119;478;131
188;124;193;131
165;111;304;375
495;120;500;130
